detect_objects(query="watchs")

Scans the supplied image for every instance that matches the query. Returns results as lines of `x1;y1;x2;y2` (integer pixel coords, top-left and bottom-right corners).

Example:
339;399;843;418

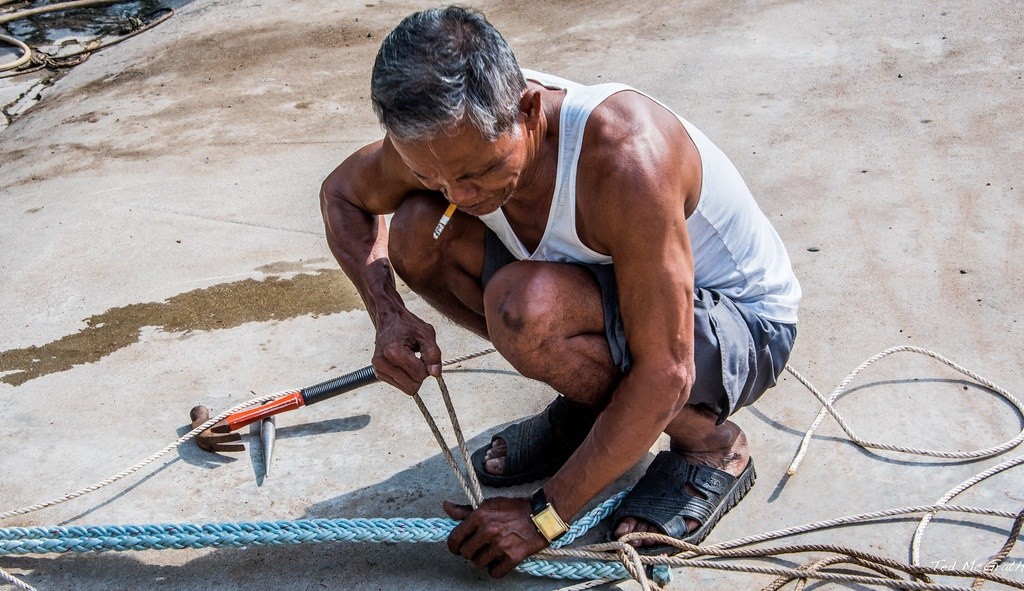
529;487;571;545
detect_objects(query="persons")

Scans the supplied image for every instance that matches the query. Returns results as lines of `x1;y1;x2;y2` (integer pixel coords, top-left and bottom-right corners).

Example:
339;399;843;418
317;6;802;579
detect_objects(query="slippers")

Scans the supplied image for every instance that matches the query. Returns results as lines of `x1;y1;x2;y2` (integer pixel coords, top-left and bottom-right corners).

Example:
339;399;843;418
605;450;758;556
471;395;601;485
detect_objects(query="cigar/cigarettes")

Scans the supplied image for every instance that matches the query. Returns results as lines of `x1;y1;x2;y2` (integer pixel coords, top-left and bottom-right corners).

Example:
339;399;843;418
432;203;457;240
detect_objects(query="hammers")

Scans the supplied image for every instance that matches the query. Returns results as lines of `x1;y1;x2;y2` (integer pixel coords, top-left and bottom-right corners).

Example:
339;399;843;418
189;365;381;451
